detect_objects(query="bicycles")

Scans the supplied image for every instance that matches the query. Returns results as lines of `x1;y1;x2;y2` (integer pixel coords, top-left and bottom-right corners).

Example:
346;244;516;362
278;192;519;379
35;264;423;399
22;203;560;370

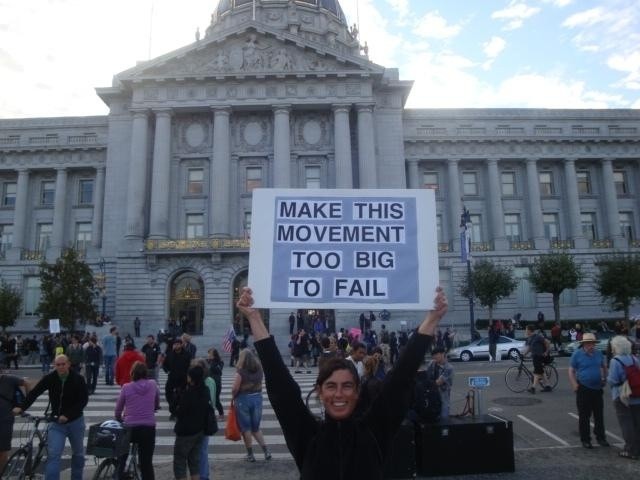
0;410;64;479
85;405;161;480
504;353;559;393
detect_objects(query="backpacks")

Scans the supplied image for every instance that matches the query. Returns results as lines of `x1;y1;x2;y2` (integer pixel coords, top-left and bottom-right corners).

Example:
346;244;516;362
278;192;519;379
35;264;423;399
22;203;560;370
610;355;640;398
415;374;442;422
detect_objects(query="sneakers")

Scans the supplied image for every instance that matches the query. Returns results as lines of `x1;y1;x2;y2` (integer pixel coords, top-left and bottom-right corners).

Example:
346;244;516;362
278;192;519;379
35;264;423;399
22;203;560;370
244;453;255;462
527;387;536;394
618;451;639;459
264;449;271;459
541;387;552;392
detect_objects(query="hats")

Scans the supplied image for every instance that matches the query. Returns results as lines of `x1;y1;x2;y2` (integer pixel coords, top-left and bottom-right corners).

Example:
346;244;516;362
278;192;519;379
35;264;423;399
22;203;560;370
578;332;600;343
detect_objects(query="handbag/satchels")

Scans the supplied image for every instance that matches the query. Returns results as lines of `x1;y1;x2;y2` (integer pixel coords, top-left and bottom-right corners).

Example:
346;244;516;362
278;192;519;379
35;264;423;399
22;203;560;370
202;406;218;436
226;406;242;441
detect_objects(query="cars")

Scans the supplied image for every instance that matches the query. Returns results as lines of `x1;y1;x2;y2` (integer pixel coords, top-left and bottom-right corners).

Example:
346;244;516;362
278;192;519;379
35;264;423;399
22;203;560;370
447;335;529;362
560;331;635;356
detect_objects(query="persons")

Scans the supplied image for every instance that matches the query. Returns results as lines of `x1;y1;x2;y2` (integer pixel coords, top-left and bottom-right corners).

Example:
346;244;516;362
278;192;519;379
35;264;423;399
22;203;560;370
487;310;638;460
472;328;481;345
2;288;459;480
201;33;315;71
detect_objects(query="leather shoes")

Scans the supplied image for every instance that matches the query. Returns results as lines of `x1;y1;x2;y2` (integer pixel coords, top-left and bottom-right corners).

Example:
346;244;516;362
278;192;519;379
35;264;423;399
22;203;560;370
597;439;609;446
583;442;592;448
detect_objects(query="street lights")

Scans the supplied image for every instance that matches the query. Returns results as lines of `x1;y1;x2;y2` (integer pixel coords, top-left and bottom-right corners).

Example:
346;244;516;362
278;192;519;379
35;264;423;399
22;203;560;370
458;204;477;337
97;254;107;324
